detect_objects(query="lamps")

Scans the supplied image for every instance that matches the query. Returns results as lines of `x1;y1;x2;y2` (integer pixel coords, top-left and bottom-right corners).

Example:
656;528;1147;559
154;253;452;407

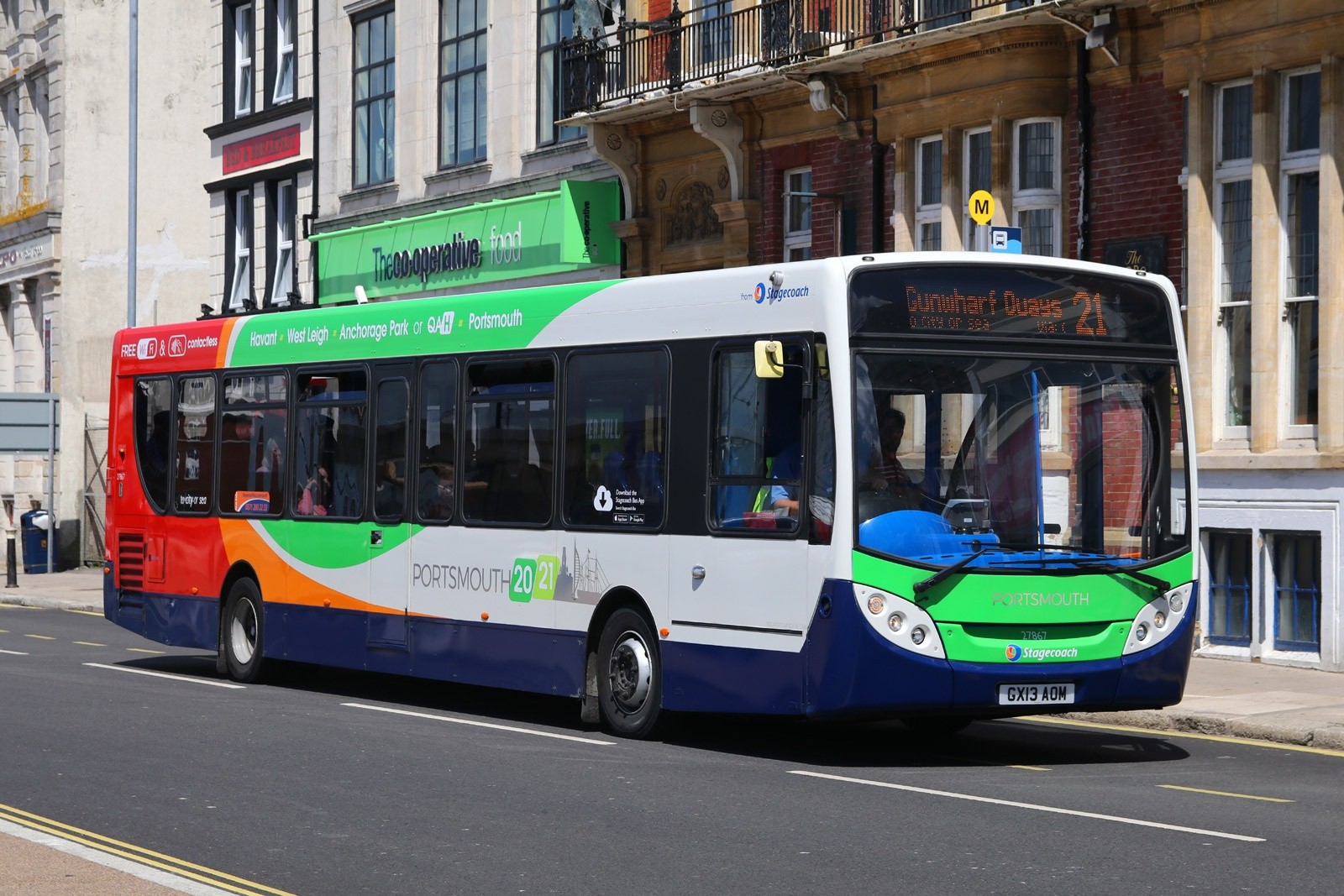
807;75;835;111
201;304;214;317
286;292;302;306
242;299;256;312
1086;4;1118;50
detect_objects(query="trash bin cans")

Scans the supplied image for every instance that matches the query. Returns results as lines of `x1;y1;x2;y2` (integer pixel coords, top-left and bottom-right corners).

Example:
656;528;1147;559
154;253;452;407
20;510;59;575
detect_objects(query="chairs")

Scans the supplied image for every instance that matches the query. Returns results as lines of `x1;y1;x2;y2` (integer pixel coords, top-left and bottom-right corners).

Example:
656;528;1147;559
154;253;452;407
598;449;801;528
145;410;525;525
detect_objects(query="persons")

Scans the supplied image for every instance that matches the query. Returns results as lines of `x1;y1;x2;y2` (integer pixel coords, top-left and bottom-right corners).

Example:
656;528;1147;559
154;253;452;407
859;406;931;512
749;451;799;520
146;410;490;521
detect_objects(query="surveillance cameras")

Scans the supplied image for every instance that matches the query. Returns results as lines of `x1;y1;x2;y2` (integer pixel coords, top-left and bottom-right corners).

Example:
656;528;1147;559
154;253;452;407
355;286;368;304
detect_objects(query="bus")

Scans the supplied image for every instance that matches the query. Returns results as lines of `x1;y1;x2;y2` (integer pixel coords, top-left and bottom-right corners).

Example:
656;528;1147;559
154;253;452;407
102;251;1203;743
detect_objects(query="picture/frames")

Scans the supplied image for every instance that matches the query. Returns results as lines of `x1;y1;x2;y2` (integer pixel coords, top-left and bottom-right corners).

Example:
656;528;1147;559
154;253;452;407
1101;235;1168;277
